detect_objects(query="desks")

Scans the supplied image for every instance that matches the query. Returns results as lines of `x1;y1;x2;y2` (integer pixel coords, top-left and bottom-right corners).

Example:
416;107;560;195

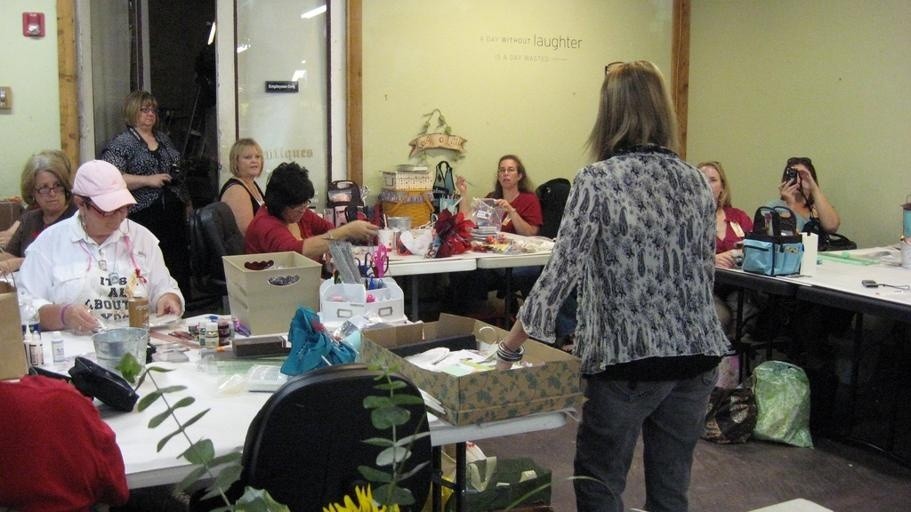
1;329;585;512
328;245;478;324
715;244;911;468
477;231;556;330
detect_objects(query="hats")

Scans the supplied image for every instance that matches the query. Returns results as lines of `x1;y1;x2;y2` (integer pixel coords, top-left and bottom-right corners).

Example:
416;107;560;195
70;159;139;213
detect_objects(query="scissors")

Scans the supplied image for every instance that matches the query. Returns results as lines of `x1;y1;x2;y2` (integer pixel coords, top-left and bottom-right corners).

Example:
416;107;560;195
353;244;390;277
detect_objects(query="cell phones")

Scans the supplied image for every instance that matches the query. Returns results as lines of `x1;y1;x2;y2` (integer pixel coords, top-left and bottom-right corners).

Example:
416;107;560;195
862;279;878;287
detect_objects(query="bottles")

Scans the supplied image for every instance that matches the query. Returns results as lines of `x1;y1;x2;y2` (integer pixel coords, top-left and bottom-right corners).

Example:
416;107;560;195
24;325;68;365
127;284;151;344
187;317;233;349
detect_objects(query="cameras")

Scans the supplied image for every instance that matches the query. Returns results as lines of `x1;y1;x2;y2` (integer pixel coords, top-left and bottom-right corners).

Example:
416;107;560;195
169;163;185;186
481;198;496;209
732;253;744;265
785;166;802;189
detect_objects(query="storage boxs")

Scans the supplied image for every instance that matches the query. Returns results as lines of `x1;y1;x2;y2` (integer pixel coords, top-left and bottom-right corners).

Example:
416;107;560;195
221;250;323;338
461;455;553;512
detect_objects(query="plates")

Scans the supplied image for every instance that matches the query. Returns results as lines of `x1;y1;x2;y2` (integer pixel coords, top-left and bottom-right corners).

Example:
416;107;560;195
101;310;177;329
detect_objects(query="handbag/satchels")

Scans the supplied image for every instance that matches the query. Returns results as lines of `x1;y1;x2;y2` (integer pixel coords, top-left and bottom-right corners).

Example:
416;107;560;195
444;456;553;511
740;205;805;278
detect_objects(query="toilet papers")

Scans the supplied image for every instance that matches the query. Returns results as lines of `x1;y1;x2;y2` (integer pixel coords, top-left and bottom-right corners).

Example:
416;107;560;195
799;232;819;278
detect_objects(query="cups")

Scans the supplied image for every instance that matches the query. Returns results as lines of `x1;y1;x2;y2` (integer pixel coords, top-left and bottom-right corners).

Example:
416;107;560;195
92;327;149;384
378;217;434;257
801;231;818;275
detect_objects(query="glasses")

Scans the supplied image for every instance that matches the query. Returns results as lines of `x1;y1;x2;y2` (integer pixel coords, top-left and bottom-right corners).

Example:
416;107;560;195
86;202;134;217
140;108;156;113
32;185;67;196
604;60;625;78
787;157;811;166
288;199;311;210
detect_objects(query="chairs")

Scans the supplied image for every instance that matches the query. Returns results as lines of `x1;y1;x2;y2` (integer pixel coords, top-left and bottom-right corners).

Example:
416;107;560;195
190;202;246;295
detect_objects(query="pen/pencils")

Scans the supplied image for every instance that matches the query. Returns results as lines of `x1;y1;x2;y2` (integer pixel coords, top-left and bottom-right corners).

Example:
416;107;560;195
249;389;278;394
364;276;383;291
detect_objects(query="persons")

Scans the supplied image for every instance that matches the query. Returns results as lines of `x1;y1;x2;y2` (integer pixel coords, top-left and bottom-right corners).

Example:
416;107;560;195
1;375;130;512
217;139;266;241
696;160;759;334
484;56;735;512
1;151;77;277
762;156;841;249
234;162;381;263
484;156;545;238
101;89;197;307
28;161;187;331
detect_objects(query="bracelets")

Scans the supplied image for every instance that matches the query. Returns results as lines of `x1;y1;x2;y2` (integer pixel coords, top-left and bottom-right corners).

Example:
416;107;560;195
495;341;528;362
324;226;335;242
57;303;70;325
